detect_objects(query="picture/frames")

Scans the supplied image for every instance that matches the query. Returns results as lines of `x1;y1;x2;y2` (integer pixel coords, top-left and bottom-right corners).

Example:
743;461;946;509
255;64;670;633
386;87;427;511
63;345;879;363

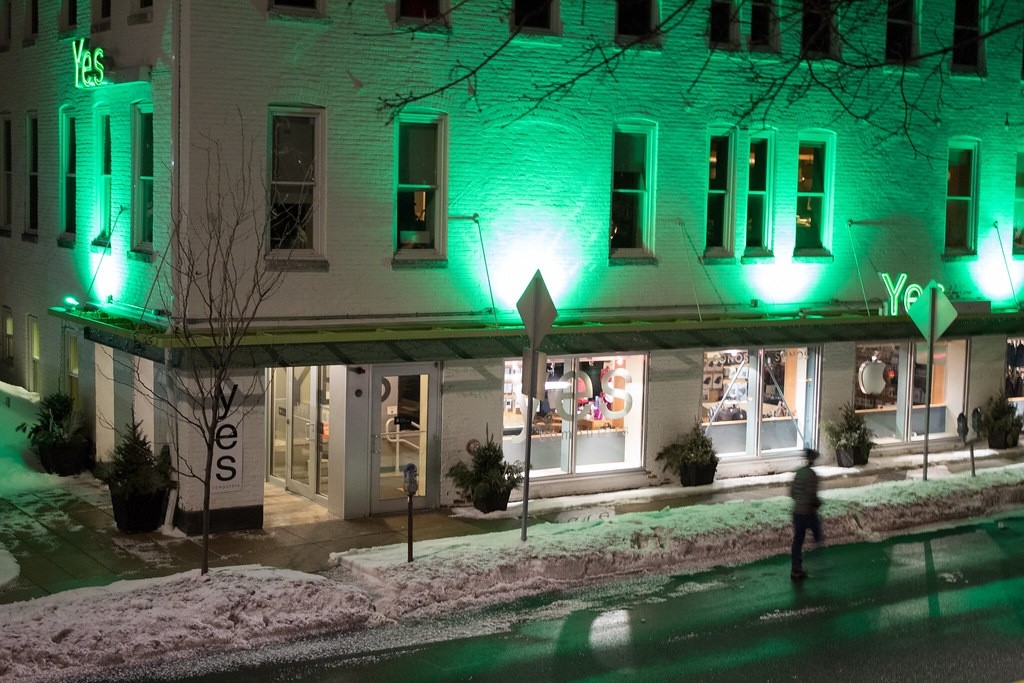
504;382;513;393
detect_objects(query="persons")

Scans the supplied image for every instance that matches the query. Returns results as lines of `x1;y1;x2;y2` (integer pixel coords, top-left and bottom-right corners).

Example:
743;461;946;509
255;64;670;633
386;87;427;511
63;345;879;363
790;448;825;583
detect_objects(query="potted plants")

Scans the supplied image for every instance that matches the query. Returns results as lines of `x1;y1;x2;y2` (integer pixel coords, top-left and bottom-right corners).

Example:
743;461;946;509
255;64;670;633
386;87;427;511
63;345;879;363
984;387;1024;450
94;401;177;535
445;421;533;513
654;414;721;486
823;400;878;467
15;377;92;477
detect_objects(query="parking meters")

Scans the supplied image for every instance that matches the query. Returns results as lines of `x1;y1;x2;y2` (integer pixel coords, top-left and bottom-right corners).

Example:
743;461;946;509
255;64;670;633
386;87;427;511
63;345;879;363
403;463;417;562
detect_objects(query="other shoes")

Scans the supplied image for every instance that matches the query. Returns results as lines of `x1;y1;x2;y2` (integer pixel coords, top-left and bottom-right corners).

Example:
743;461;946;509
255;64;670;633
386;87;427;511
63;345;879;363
789;570;809;580
